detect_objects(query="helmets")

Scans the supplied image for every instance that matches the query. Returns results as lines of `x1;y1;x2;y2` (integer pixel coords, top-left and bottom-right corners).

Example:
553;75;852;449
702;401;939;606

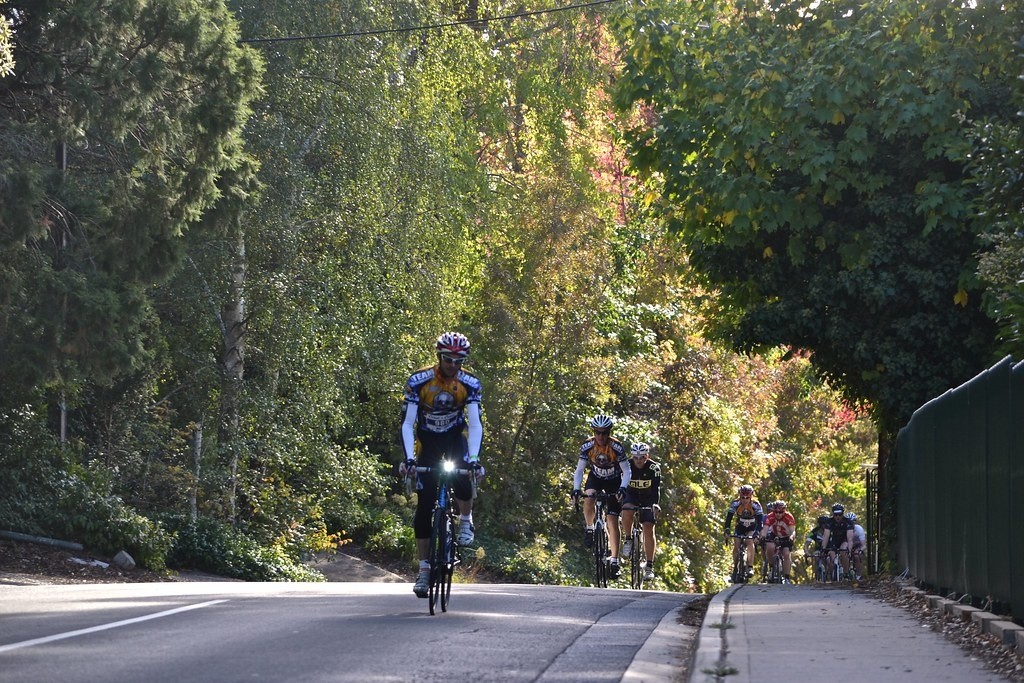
773;501;787;511
845;512;857;521
766;502;773;510
591;415;612;429
818;516;829;524
832;504;844;513
630;442;649;454
739;485;754;496
436;332;470;356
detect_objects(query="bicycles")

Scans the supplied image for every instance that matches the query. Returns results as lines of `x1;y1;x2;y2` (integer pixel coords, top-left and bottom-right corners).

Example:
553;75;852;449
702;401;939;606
760;534;862;587
725;534;755;585
402;460;478;616
574;489;611;588
620;506;658;590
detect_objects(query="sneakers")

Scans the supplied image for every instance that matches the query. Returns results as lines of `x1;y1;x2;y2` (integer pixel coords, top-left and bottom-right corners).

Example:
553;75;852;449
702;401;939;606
456;519;476;545
413;568;430;593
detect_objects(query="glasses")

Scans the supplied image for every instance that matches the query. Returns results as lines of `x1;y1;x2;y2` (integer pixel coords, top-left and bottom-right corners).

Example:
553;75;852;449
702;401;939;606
595;430;609;435
741;496;750;499
835;514;842;516
775;511;783;514
441;353;465;365
633;456;644;460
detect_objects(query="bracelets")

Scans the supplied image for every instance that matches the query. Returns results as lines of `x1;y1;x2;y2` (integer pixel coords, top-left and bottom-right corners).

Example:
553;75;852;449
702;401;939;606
469;456;480;459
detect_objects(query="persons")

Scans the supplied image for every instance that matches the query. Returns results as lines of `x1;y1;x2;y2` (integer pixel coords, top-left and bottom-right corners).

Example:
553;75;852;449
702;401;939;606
572;415;631;574
400;332;483;594
846;512;866;579
760;500;796;584
725;485;763;582
821;503;853;582
622;442;662;580
804;515;829;580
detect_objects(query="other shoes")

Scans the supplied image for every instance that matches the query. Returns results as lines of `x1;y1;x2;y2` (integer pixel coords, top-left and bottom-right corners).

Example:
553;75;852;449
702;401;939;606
585;529;593;547
762;566;767;574
644;567;655;580
611;563;622;577
731;574;736;581
623;540;633;557
747;565;754;575
785;578;790;586
